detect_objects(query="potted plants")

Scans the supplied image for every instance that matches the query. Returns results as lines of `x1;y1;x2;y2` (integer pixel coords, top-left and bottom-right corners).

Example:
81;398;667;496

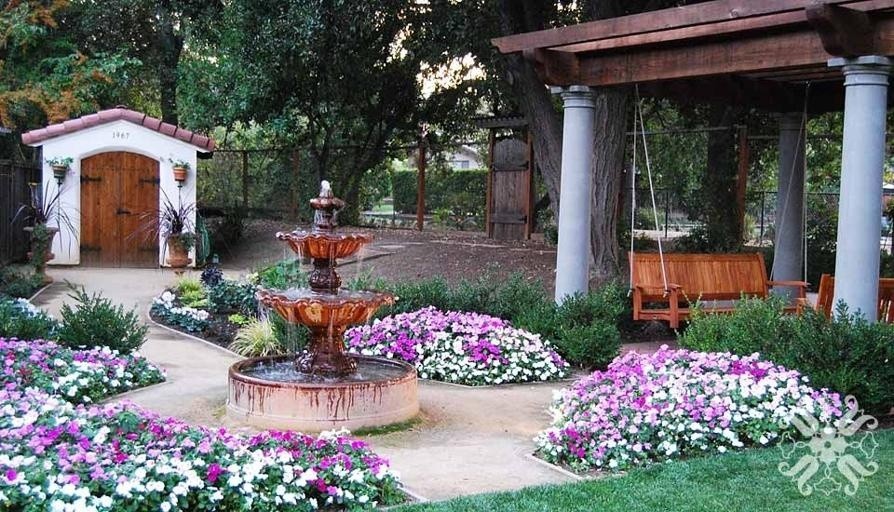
44;156;74;178
169;158;192;181
124;185;204;288
8;180;104;285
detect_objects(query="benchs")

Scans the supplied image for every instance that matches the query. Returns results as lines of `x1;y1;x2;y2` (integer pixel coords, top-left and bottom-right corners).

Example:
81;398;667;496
628;249;894;328
